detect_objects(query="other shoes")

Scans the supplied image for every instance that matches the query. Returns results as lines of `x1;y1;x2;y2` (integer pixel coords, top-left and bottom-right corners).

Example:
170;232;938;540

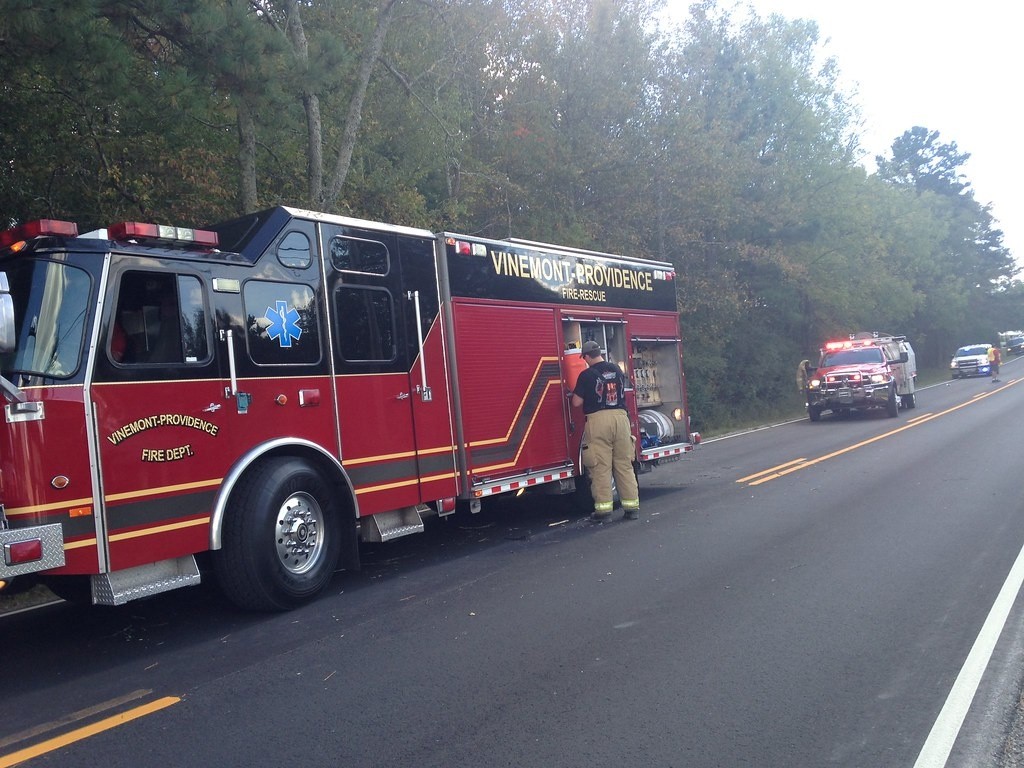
622;510;640;520
590;512;613;524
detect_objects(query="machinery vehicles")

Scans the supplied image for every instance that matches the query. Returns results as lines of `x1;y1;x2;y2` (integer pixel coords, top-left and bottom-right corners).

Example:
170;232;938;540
796;331;918;421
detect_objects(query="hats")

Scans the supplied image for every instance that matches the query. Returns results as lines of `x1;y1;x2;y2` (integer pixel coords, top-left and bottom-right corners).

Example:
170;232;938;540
579;341;601;358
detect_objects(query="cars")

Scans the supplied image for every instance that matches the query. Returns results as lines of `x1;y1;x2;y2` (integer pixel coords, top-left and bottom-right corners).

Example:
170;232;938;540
1003;338;1024;356
950;344;993;378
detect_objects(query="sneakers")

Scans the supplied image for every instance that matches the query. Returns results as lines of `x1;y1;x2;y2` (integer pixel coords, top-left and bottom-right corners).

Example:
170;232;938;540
991;377;1001;383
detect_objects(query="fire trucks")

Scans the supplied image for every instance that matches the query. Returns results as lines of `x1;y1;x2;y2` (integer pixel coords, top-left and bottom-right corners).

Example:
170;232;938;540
0;205;702;609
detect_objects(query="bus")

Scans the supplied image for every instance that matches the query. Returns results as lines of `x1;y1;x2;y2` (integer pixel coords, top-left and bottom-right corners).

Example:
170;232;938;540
998;331;1022;346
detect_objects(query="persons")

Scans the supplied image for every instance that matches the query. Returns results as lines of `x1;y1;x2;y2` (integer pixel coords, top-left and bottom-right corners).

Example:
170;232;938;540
572;340;639;523
987;343;1001;382
